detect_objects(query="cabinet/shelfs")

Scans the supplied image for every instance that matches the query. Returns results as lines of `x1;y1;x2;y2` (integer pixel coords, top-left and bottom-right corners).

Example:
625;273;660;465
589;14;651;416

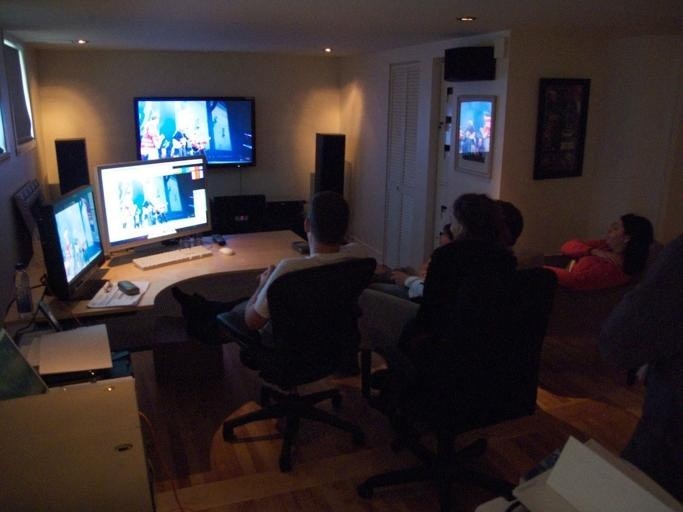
0;322;156;511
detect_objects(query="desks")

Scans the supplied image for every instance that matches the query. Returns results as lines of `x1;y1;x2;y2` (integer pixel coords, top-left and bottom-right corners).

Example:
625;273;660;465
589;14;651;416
3;229;311;324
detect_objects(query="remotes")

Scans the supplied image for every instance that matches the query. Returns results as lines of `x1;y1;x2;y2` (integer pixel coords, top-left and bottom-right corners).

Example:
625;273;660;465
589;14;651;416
213;234;226;245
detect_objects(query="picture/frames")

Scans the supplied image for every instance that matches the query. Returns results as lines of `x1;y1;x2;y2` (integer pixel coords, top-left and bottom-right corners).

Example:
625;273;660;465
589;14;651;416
533;77;592;181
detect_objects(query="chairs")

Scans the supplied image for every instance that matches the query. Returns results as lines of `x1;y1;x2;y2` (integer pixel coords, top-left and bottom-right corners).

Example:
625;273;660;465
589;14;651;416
353;268;557;510
533;236;665;397
225;257;378;471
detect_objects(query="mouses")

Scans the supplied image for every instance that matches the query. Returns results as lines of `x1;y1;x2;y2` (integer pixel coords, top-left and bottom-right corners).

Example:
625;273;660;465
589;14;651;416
219;247;234;256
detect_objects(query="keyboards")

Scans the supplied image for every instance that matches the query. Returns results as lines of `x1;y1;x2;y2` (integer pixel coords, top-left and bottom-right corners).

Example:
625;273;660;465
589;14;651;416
132;245;212;269
94;281;151;307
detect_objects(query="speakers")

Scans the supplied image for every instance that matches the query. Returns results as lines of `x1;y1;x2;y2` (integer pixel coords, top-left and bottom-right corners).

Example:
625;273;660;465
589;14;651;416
55;140;89;194
315;134;345;194
444;46;495;81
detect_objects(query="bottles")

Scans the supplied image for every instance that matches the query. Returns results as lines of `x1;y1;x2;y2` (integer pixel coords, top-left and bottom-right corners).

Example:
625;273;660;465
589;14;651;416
13;262;33;318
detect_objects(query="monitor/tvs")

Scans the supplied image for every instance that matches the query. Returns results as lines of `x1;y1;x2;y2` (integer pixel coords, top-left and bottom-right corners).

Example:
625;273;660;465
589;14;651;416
92;155;212;255
36;184;107;301
132;95;255;169
458;99;491;164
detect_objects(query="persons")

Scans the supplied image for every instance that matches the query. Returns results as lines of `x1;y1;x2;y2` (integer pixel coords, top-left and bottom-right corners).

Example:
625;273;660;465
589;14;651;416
488;199;523;274
543;213;653;289
229;191;366;347
367;224;453;306
605;234;683;503
399;193;503;363
